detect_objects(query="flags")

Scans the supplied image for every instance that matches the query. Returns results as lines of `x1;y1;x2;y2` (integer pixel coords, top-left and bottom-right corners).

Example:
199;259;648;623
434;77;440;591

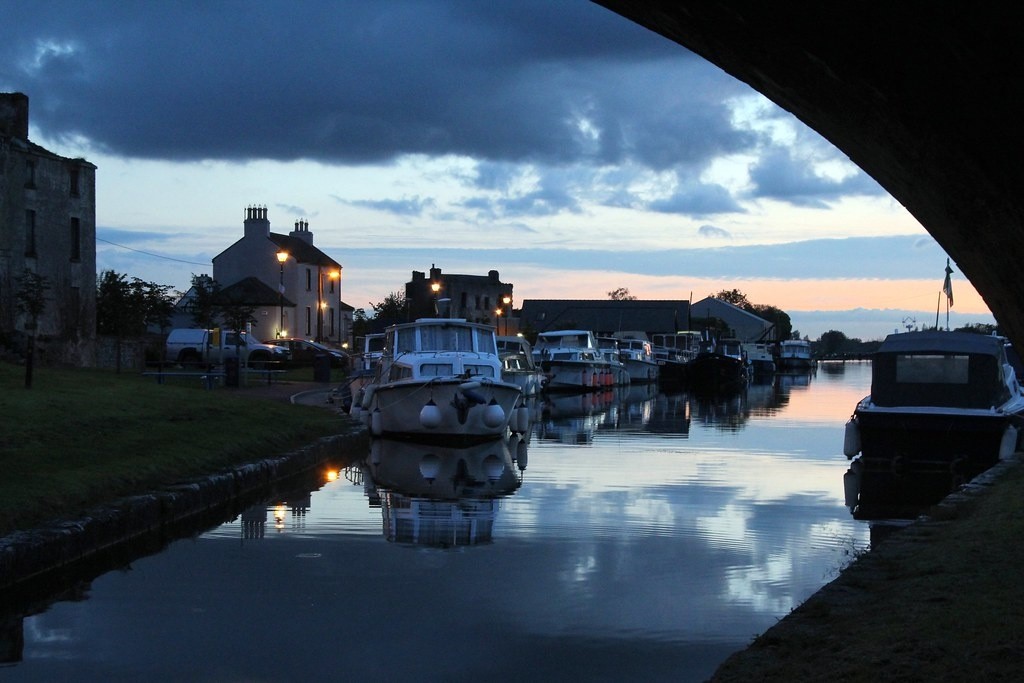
943;267;954;307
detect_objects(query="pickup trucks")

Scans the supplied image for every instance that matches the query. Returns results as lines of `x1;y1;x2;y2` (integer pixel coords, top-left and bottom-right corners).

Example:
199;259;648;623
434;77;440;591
166;329;292;371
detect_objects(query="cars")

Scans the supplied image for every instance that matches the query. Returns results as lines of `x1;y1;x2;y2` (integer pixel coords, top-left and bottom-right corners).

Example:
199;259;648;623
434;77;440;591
262;338;350;369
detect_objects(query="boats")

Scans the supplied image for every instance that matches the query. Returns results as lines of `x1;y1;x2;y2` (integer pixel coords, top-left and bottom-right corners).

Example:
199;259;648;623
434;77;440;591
366;432;520;550
362;317;521;445
621;333;753;382
852;329;1024;525
594;337;627;384
775;340;816;371
747;344;776;375
346;333;385;401
537;388;614;446
532;330;612;386
494;336;549;396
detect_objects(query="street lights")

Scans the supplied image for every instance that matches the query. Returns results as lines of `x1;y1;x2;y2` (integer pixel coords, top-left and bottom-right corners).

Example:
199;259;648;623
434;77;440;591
503;296;509;336
496;310;500;336
276;249;288;338
405;296;412;323
431;282;440;318
317;266;339;344
